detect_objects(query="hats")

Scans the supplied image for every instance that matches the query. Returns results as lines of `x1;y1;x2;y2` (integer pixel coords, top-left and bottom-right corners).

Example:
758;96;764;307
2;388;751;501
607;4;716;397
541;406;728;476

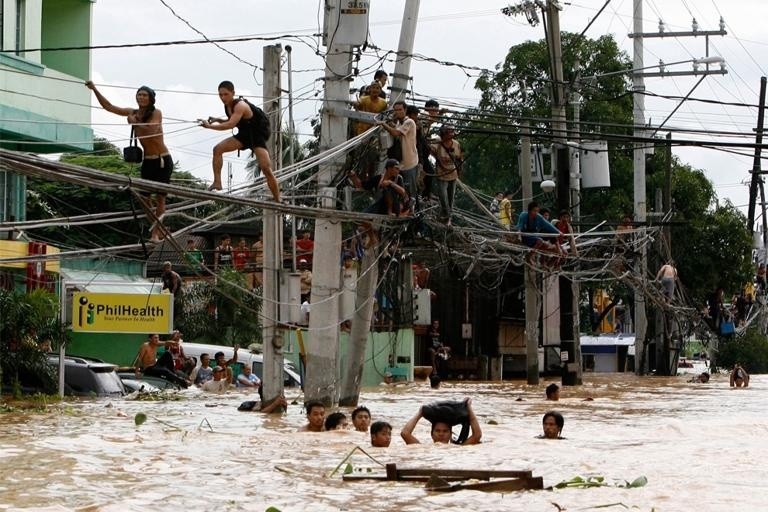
371;81;382;87
385;159;404;167
212;366;226;371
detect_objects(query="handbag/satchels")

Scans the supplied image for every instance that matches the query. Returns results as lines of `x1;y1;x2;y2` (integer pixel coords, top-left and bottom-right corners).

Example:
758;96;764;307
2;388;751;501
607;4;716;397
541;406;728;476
124;147;142;163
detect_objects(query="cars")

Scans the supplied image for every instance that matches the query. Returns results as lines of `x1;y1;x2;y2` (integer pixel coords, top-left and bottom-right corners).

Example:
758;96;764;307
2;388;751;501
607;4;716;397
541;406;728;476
111;364;180;398
676;361;696;376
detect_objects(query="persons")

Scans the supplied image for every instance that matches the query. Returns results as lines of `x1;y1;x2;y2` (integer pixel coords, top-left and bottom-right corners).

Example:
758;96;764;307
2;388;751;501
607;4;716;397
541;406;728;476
4;323;53;355
613;317;622;332
708;262;766;329
615;217;633;243
546;384;560;402
653;259;679;306
729;363;750;388
400;397;481;444
252;384;287;413
297;402;392;447
534;410;565;440
700;371;710;385
492;189;585;273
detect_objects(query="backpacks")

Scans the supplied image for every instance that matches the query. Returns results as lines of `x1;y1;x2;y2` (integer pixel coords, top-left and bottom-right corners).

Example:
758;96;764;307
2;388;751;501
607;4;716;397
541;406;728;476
423;401;468;424
232;99;270;140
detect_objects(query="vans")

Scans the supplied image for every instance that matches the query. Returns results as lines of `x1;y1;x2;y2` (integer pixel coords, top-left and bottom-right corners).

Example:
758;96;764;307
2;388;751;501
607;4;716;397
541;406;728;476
181;341;302;390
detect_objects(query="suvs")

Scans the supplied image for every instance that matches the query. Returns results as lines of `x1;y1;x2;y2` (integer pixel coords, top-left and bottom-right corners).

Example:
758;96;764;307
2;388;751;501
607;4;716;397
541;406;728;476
6;349;127;399
